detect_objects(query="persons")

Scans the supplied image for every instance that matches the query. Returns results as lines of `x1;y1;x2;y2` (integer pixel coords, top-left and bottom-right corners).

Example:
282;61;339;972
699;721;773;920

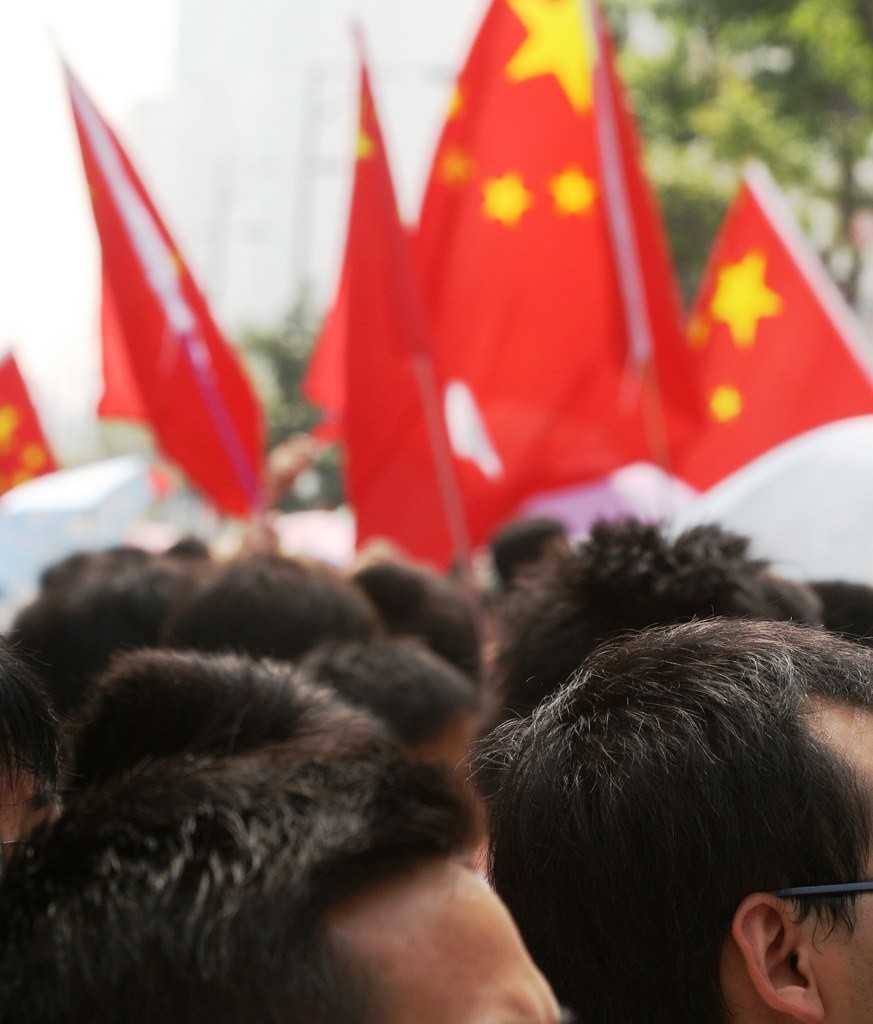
0;735;566;1024
486;518;774;749
305;639;481;783
0;518;873;855
483;622;873;1023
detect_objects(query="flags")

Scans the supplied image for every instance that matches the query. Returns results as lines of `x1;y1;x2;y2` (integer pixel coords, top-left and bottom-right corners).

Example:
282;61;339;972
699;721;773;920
66;98;271;522
309;0;704;546
341;77;466;578
1;349;55;495
651;192;872;493
